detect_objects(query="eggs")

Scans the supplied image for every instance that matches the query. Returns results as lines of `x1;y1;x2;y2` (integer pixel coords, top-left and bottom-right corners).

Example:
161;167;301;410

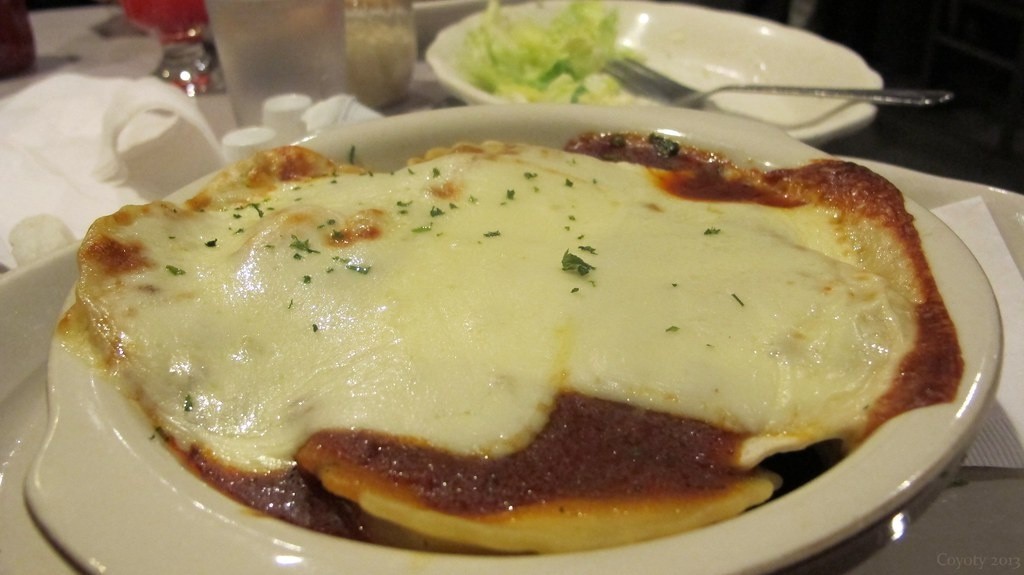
55;128;964;554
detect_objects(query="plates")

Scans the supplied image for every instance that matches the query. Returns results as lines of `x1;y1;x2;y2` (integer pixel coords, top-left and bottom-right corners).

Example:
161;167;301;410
19;94;1010;575
424;3;883;151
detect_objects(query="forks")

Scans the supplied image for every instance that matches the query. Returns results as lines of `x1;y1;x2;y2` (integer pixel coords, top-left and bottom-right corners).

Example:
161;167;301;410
602;58;954;109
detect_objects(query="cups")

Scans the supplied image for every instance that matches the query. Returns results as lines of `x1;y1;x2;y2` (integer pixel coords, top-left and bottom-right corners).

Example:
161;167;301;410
204;0;352;133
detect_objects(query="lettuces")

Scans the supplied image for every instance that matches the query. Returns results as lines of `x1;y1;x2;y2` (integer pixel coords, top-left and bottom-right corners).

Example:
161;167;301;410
461;1;652;106
